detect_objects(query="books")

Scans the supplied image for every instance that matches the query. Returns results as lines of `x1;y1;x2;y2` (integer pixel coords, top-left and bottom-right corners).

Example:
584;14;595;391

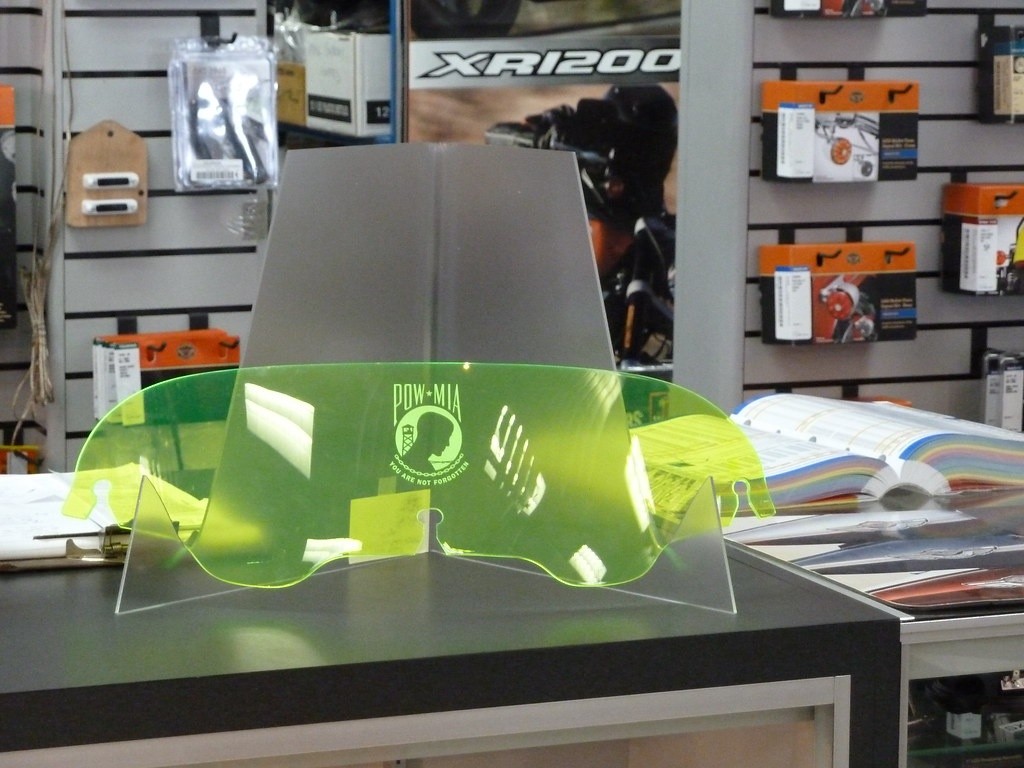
629;391;1024;514
0;464;262;571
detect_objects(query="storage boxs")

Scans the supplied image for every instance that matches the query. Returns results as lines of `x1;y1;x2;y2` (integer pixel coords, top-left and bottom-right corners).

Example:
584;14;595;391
769;0;927;20
758;241;917;347
272;18;392;139
975;25;1024;126
761;79;919;183
939;182;1024;298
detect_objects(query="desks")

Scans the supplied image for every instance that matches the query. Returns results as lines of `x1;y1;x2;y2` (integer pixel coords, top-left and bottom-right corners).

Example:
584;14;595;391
0;491;901;768
650;507;1024;768
